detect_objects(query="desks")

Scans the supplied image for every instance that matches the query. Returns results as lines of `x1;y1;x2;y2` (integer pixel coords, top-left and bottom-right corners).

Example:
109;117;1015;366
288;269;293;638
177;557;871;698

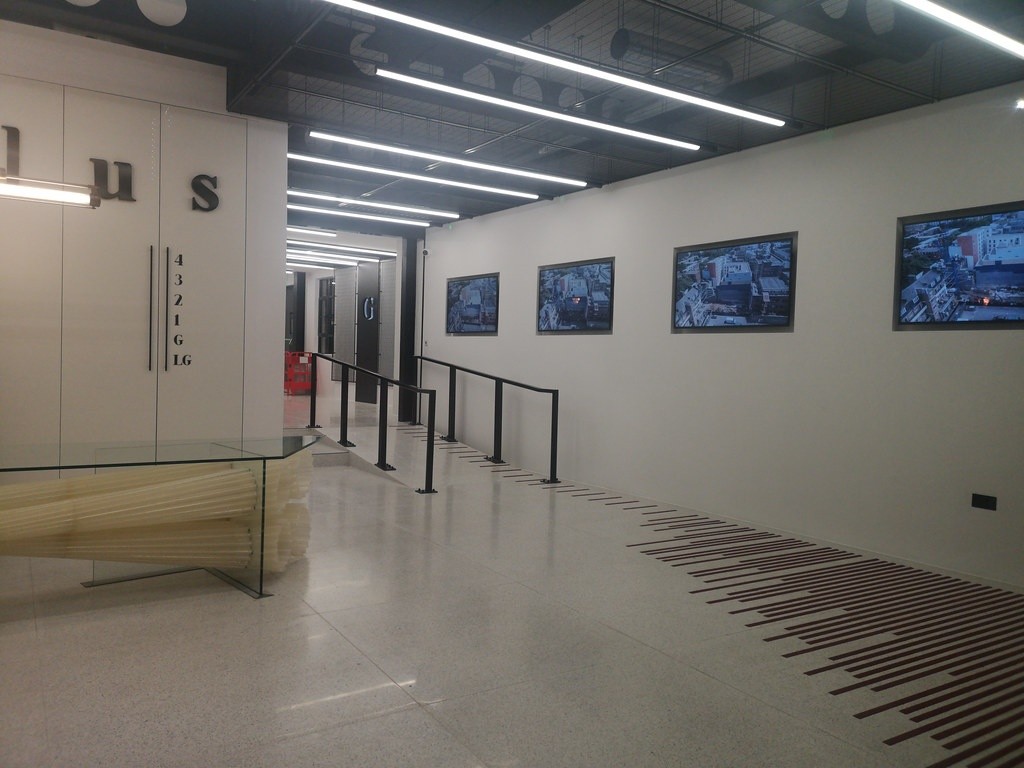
0;435;327;600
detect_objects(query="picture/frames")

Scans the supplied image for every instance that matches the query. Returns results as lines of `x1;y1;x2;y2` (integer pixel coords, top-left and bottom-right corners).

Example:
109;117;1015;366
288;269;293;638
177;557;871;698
670;230;799;333
893;200;1024;331
536;256;616;336
445;272;500;333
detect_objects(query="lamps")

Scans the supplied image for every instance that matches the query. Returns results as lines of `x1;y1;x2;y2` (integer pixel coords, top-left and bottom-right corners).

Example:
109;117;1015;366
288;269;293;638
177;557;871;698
0;168;101;210
323;0;805;157
286;132;602;275
897;0;1024;59
